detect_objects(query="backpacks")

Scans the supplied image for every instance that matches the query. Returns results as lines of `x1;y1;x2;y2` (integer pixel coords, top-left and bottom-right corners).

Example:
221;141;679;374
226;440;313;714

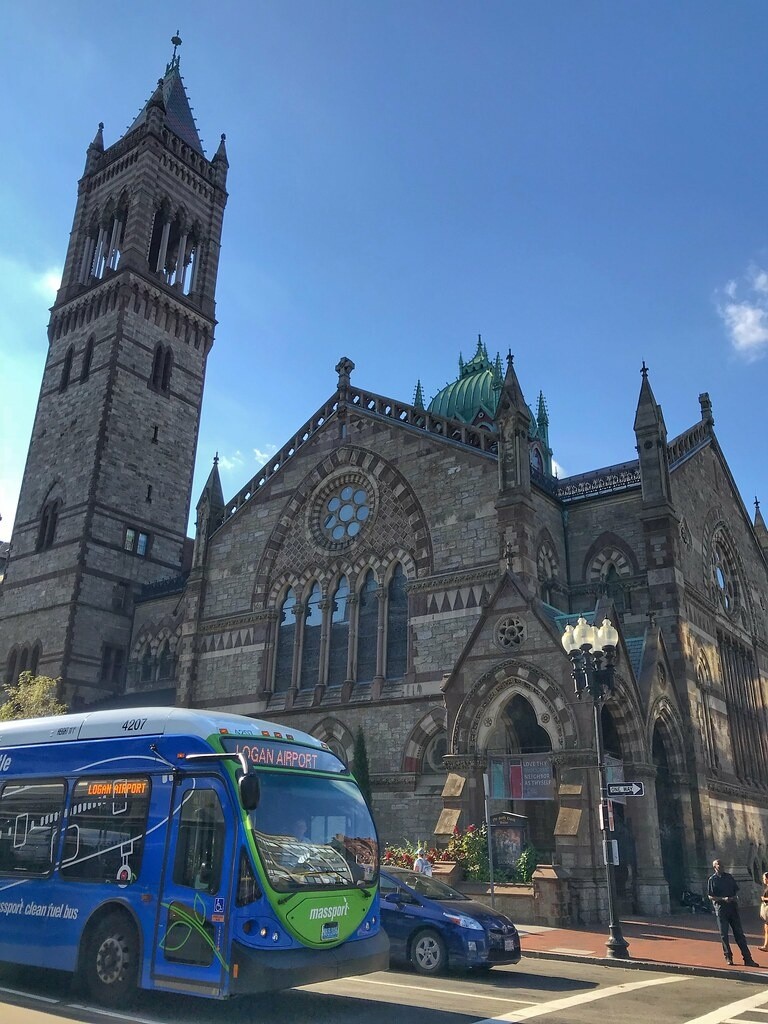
419;859;432;877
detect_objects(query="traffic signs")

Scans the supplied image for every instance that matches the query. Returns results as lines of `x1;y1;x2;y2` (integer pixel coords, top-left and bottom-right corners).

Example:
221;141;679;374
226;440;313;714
607;781;646;796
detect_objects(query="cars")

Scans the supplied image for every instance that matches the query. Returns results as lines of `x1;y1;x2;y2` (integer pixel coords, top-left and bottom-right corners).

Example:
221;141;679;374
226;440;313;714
360;866;523;979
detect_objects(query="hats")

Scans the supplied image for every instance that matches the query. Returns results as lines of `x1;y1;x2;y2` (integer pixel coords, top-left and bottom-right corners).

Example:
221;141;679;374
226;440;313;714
415;848;425;855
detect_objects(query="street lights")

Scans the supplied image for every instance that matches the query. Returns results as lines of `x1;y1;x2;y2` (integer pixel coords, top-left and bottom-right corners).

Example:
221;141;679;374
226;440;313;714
561;612;632;960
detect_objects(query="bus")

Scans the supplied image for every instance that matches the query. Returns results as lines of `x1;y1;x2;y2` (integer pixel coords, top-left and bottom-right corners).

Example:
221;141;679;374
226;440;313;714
0;706;378;1008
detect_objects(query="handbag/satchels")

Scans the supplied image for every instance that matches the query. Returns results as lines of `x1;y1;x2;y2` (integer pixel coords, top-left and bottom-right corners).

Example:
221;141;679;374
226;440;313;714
760;902;768;924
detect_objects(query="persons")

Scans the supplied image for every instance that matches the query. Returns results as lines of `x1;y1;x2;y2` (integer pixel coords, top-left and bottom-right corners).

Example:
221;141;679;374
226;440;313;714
758;871;768;953
280;818;314;871
413;848;432;896
707;857;761;967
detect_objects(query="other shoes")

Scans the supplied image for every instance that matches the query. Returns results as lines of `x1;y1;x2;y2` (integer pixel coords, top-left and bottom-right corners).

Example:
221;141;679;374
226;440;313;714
758;946;768;952
725;957;733;965
744;959;759;966
